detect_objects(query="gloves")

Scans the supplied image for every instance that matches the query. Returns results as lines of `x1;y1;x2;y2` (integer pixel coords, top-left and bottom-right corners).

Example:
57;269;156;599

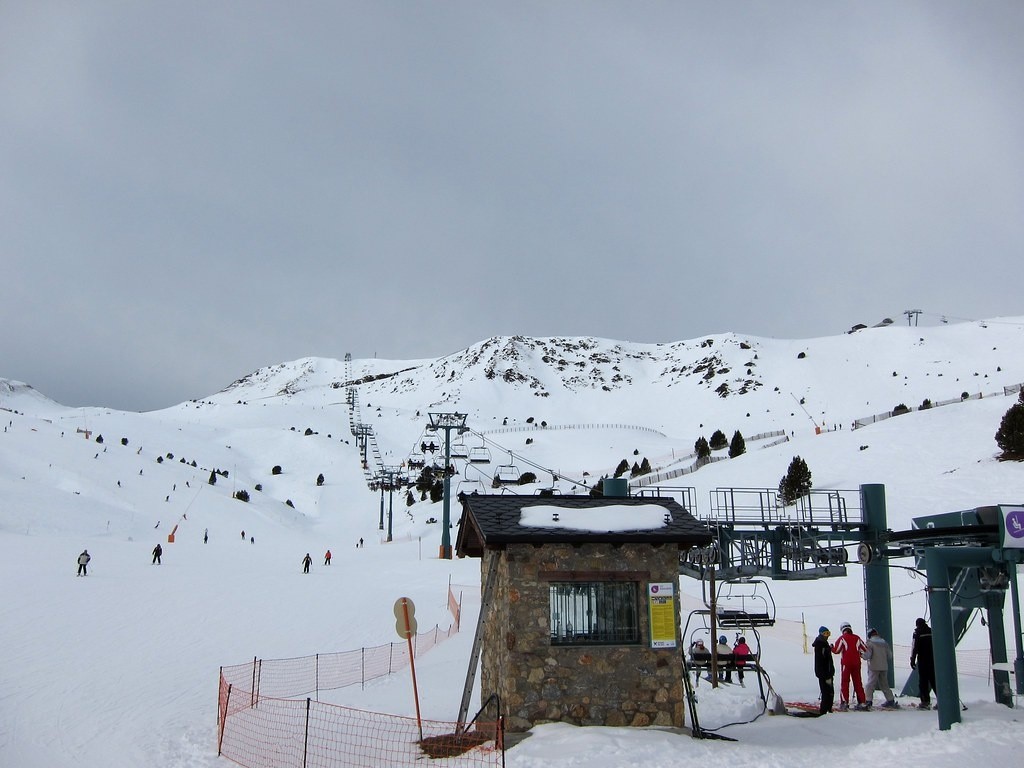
910;657;917;670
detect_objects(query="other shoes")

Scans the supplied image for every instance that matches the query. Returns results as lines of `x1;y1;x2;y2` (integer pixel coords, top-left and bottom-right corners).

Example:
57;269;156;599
838;704;849;711
915;704;930;711
855;703;867;710
880;700;895;707
724;677;732;683
866;700;872;707
705;673;712;683
933;704;937;710
719;673;724;680
739;679;743;683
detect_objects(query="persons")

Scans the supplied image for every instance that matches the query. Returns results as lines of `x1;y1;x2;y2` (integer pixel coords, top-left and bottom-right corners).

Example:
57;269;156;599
369;438;482;506
829;621;870;710
715;634;734;684
77;549;91;576
909;618;937;710
691;639;712;688
116;467;368;579
733;637;751;683
861;628;899;707
811;625;835;716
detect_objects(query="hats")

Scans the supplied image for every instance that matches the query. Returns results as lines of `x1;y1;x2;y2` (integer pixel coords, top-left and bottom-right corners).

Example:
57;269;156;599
819;626;830;637
739;637;745;644
915;618;926;626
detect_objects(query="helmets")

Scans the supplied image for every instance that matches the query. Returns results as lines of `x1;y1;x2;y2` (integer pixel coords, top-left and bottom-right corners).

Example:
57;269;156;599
840;622;850;631
719;635;728;644
867;628;878;636
697;639;703;646
